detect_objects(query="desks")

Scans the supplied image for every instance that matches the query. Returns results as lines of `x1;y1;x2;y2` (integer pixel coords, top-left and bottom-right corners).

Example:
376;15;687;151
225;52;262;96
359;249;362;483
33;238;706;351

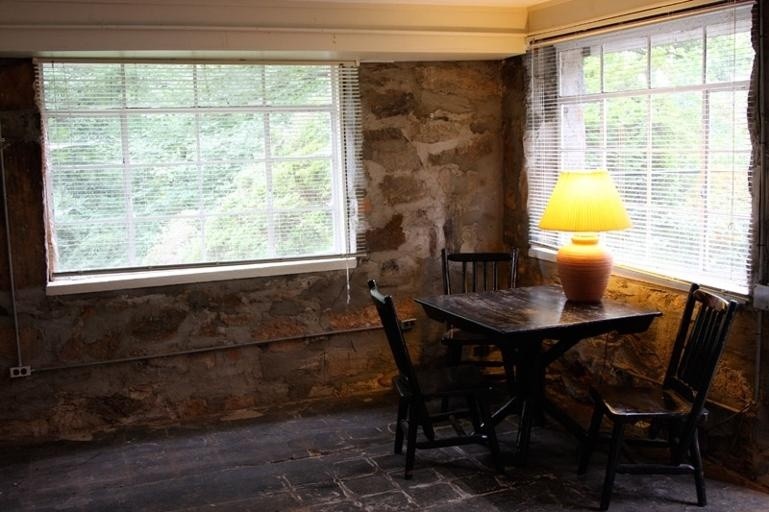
414;283;663;468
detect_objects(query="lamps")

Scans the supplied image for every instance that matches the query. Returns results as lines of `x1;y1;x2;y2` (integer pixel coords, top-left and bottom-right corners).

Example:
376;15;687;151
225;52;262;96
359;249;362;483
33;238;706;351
539;170;633;300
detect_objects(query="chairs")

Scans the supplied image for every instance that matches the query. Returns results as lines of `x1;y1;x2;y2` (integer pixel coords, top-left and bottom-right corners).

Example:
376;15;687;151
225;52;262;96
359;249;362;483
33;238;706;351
581;284;735;511
368;280;506;480
441;248;519;412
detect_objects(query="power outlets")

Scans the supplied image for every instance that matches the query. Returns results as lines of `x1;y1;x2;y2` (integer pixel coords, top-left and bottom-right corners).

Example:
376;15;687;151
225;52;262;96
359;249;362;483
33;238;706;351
10;366;31;378
